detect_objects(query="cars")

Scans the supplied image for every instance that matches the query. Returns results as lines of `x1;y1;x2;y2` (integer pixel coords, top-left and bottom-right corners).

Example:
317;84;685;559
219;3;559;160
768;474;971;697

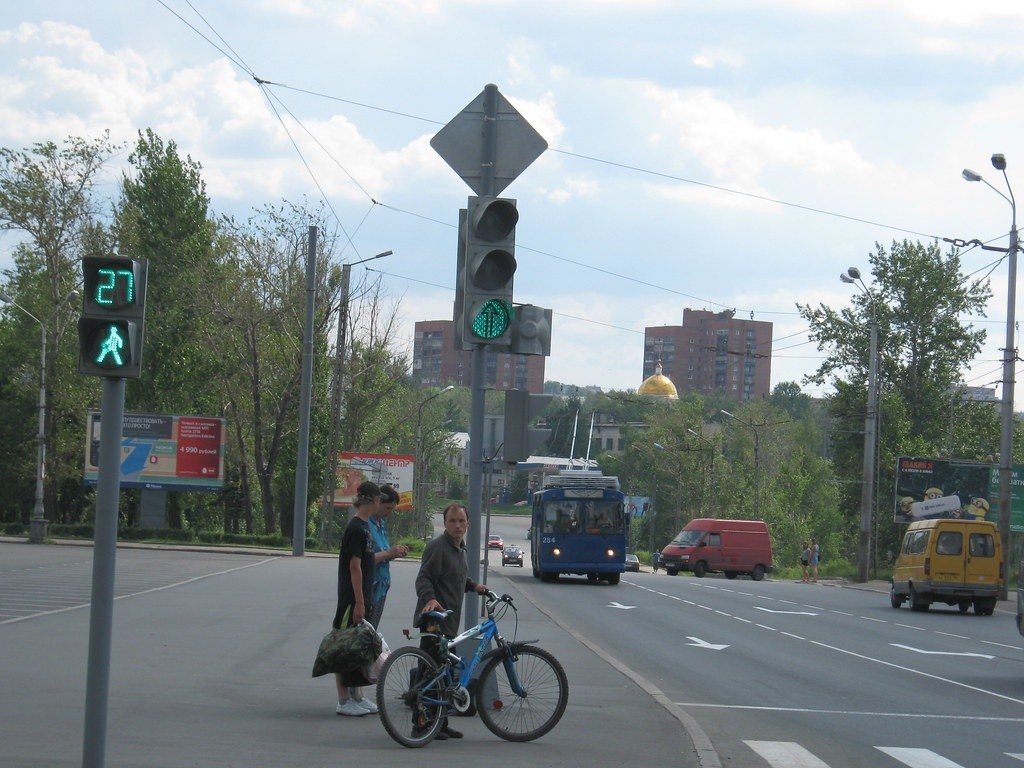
501;546;525;568
624;552;640;572
487;535;503;550
527;527;532;540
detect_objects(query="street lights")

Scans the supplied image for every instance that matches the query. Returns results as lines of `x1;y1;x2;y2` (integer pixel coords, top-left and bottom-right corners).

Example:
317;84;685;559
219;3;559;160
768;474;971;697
326;249;393;547
840;265;879;581
414;384;455;542
653;442;683;517
0;289;49;543
961;153;1019;599
719;409;759;517
688;428;714;517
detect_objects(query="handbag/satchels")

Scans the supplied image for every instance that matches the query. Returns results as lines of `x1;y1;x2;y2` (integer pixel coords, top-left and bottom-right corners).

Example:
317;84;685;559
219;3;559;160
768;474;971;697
357;618;392;684
312;603;382;678
818;555;820;561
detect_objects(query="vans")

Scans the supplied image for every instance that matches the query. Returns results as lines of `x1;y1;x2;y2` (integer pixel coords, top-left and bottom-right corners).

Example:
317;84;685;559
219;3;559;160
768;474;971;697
890;519;1005;615
658;519;774;581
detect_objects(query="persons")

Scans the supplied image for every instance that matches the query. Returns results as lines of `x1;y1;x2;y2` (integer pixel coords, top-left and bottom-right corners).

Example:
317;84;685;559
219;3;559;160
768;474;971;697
623;501;649;516
800;542;810;584
412;503;490;739
559;504;613;533
810;538;821;582
652;549;662;574
363;485;411;633
335;480;380;716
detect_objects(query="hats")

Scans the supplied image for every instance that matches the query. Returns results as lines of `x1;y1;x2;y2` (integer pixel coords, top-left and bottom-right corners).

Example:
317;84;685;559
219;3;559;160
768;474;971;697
357;481;389;500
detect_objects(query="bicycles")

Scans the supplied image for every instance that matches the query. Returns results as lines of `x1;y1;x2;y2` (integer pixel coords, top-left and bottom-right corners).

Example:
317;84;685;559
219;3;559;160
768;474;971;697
374;586;569;748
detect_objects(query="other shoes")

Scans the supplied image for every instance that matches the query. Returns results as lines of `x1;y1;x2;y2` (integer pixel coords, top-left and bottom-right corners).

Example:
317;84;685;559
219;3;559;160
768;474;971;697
411;728;449;740
441;727;463;738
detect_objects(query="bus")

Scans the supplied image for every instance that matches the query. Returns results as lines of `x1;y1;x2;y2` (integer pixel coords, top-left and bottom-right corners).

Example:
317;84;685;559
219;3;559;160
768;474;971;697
529;471;630;583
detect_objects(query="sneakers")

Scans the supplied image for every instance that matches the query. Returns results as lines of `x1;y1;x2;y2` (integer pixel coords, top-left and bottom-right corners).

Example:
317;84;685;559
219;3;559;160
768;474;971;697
335;697;370;716
352;697;379;713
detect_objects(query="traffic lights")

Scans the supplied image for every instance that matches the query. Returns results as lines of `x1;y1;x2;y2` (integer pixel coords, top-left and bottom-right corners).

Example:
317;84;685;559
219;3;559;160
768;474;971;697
78;254;151;379
464;196;520;346
